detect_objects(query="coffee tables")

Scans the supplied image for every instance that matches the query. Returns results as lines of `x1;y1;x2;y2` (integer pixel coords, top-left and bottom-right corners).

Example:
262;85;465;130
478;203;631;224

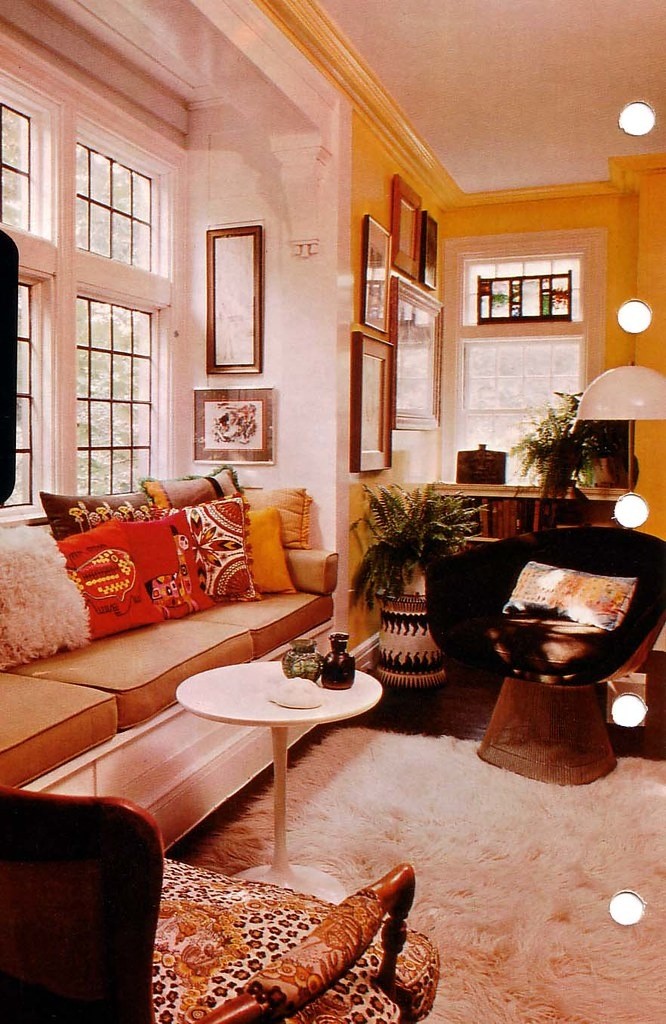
176;653;385;909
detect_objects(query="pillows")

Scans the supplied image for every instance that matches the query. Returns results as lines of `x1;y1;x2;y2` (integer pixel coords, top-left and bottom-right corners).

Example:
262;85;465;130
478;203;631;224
0;524;91;673
100;508;217;626
159;491;263;604
246;507;298;597
140;466;241;508
56;519;160;651
36;489;155;537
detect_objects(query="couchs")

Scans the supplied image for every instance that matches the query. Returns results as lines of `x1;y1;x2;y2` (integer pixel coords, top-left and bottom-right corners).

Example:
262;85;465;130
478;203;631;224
0;464;342;853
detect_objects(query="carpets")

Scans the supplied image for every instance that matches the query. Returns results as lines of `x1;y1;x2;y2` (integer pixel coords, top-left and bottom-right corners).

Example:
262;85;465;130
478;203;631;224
182;720;666;1024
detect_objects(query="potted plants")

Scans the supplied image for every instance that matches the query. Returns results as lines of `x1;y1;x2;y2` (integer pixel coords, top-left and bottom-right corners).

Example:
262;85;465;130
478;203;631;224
563;417;628;487
345;479;489;691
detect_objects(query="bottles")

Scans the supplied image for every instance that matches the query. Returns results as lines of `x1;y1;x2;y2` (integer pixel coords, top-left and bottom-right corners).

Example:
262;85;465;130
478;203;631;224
281;638;323;683
321;631;356;691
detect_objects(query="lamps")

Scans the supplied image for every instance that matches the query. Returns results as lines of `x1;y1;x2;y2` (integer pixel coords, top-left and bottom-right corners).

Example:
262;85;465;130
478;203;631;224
575;360;666;493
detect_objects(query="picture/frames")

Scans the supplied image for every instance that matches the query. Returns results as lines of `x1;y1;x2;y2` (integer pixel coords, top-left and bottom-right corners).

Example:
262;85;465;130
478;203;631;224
389;173;422;280
192;384;277;466
360;213;393;336
423;211;441;292
350;330;397;474
390;273;443;431
204;224;266;376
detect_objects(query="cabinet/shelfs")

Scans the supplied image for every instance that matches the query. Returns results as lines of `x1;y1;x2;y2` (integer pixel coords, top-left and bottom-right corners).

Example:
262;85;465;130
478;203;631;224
427;483;629;547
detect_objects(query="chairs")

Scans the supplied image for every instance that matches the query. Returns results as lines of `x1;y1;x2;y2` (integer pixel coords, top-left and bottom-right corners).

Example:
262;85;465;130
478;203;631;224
423;524;666;789
0;784;441;1024
